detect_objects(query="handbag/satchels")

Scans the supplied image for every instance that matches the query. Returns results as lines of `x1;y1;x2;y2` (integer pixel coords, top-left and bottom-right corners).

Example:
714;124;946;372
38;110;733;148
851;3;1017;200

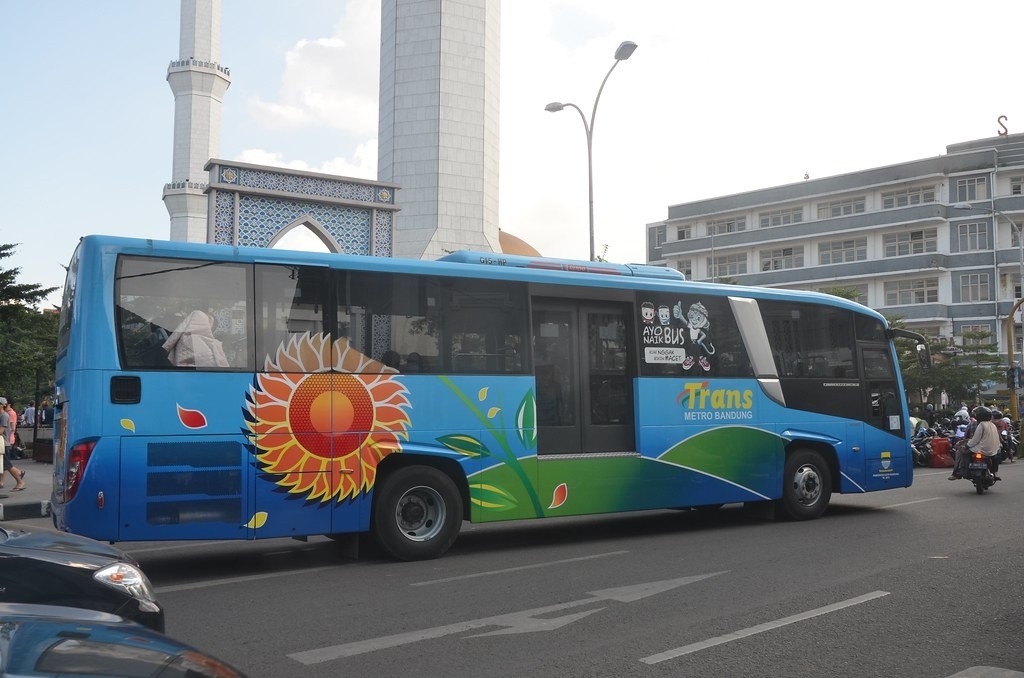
9;430;28;460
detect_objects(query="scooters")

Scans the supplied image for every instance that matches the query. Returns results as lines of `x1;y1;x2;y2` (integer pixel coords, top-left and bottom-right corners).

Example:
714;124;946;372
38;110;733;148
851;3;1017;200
968;452;997;495
908;420;1022;467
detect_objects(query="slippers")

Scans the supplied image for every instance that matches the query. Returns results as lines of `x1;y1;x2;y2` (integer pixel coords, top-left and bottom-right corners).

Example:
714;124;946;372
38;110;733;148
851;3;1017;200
0;484;5;489
10;486;27;491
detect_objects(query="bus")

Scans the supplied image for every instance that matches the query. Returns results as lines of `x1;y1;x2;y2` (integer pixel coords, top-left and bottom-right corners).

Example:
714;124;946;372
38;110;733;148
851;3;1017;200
49;234;935;564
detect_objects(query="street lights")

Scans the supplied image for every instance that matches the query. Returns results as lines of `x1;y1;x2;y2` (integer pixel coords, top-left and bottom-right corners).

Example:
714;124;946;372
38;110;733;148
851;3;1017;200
954;202;1024;387
545;40;640;263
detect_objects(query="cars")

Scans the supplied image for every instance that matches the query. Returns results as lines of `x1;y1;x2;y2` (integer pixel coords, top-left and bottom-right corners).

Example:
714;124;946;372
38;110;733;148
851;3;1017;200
1;519;166;637
1;601;247;678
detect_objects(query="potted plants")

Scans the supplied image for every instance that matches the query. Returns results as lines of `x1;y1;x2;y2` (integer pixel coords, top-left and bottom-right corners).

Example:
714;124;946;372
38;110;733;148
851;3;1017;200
34;437;54;463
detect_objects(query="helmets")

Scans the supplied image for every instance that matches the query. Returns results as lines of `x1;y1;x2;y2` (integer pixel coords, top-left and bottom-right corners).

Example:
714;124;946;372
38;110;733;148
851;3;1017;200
992;411;1003;419
954;406;970;421
925;403;934;411
971;406;992;421
1003;413;1012;418
943;417;950;426
925;427;938;437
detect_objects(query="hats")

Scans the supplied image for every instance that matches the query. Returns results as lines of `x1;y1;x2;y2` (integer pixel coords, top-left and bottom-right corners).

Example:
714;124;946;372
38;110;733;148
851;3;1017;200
28;400;35;405
0;397;8;407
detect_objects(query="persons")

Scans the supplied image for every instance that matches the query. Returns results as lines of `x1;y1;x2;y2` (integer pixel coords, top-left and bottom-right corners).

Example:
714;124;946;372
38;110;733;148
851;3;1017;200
381;350;400;370
910;404;937;428
947;405;1022;480
0;397;54;492
407;352;424;373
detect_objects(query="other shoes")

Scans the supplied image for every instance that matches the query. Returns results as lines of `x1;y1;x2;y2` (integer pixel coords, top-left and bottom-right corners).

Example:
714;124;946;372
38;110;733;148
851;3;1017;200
993;477;1001;481
20;469;26;481
947;474;962;481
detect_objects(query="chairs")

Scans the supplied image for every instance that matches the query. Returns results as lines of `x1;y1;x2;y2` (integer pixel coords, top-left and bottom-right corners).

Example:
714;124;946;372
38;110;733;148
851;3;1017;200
380;349;473;371
154;328;171;366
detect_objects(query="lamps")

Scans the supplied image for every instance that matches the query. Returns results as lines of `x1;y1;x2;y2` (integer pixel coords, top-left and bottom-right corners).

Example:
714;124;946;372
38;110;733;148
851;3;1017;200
804;172;810;181
